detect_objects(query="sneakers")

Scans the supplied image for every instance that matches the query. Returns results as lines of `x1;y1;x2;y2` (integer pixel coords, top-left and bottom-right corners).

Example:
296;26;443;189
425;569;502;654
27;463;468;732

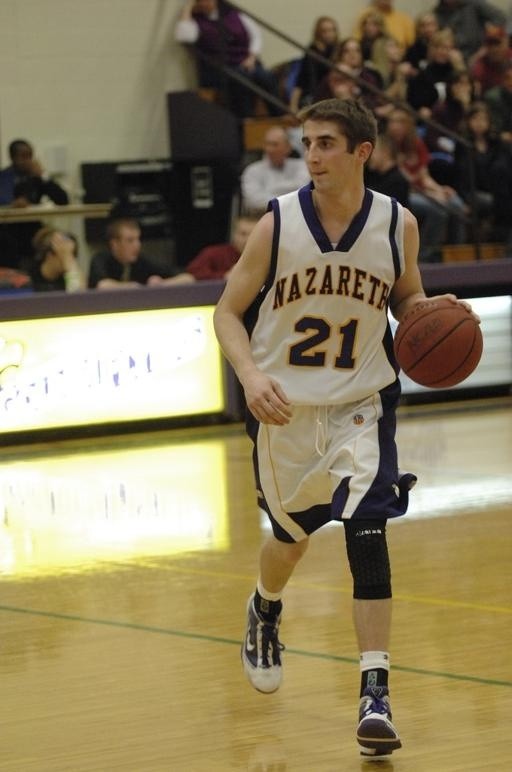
358;691;404;762
238;589;282;695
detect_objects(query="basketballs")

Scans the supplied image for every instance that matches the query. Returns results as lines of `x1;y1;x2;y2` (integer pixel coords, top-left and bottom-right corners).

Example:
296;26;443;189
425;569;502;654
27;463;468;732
393;299;483;388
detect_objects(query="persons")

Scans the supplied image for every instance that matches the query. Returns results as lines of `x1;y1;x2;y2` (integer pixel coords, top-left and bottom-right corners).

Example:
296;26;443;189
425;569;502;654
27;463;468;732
28;227;88;294
188;210;266;278
240;121;311;212
287;1;511;263
174;0;288;116
0;139;69;208
213;96;481;761
88;215;196;291
